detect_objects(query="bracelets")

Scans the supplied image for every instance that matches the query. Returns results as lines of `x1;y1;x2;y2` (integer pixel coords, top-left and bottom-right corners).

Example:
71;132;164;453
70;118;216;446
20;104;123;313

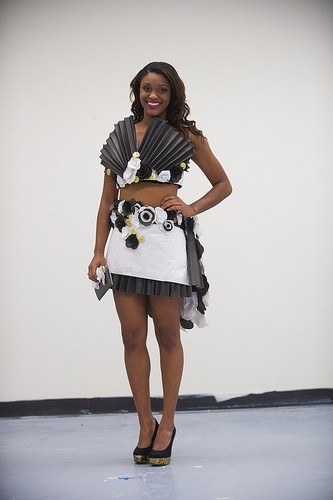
190;204;197;215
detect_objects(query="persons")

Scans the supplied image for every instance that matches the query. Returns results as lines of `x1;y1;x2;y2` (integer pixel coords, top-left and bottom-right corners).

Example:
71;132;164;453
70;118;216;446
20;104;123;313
86;62;234;464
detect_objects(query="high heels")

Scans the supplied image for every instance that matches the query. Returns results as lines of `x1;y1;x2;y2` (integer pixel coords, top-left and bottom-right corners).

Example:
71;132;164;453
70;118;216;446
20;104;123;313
149;426;176;465
134;417;159;463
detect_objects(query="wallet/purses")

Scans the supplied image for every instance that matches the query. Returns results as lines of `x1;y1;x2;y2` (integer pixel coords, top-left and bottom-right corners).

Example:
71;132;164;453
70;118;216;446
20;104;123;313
93;265;113;301
